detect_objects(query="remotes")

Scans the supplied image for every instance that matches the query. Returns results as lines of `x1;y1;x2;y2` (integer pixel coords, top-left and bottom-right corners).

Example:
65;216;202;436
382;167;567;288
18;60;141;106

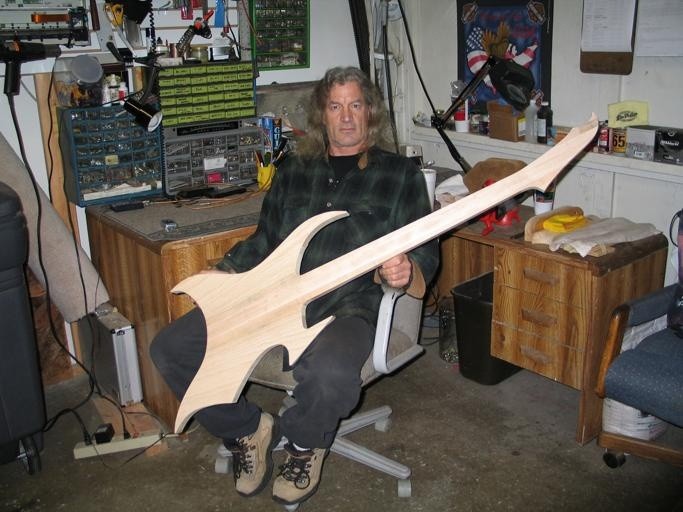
109;198;145;212
207;185;247;199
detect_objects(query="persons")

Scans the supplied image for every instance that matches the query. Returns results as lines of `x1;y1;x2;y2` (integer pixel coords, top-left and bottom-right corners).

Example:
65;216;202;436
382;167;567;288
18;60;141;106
148;64;441;507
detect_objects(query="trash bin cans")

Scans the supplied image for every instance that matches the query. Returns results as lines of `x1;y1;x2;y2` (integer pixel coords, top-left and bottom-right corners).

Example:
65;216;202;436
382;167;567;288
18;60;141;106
450;270;524;386
0;180;47;475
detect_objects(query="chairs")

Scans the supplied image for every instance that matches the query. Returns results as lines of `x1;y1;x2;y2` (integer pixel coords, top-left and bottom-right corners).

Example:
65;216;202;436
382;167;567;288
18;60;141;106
597;213;683;469
188;165;438;511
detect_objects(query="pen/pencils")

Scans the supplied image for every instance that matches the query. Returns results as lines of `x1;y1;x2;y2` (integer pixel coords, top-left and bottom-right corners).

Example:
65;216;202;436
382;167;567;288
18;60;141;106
253;138;290;167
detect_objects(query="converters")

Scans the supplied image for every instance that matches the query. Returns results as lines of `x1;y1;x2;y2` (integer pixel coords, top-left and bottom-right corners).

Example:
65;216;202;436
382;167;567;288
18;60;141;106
178;185;216;199
94;422;114;441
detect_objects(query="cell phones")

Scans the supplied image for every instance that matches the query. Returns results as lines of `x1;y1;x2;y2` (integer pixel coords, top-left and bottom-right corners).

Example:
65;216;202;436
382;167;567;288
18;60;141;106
159;219;179;234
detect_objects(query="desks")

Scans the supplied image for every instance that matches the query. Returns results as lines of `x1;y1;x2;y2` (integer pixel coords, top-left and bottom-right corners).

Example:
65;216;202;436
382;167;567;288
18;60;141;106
85;168;667;434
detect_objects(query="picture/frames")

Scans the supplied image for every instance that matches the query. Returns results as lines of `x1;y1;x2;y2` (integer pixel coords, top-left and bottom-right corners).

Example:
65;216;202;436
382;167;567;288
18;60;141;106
458;0;551;113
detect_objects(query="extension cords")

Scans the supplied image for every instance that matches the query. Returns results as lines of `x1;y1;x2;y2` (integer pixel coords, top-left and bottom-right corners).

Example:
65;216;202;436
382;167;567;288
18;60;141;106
73;428;162;459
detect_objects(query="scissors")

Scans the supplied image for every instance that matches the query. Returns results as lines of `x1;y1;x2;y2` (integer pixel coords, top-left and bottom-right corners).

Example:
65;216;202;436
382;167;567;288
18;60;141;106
104;4;137;57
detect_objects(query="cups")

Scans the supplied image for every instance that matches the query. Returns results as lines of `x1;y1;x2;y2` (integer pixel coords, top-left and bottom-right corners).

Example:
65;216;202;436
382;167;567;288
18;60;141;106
152;43;207;67
256;167;273;191
261;117;283;162
532;179;555;215
419;168;436;213
412;96;489;136
546;127;557;145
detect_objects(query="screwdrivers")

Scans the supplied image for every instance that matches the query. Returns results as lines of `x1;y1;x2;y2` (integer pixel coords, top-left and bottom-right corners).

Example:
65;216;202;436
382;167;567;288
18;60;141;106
108;200;190;212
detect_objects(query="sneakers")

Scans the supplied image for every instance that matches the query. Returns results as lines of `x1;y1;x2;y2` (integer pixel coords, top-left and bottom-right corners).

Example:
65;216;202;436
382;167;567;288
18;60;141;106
272;438;329;504
222;412;283;497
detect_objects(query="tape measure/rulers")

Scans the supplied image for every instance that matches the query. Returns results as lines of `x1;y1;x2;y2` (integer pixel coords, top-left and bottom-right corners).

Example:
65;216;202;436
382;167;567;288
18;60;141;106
272;117;282;154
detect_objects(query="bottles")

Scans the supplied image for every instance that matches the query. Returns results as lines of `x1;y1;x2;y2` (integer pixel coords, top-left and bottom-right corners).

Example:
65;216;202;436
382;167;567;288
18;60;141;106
524;100;538;144
101;74;130;108
536;103;552;143
593;120;609;154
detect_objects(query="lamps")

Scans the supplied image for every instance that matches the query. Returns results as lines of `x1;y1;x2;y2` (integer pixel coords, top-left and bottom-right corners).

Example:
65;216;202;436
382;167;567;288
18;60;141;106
119;93;164;137
